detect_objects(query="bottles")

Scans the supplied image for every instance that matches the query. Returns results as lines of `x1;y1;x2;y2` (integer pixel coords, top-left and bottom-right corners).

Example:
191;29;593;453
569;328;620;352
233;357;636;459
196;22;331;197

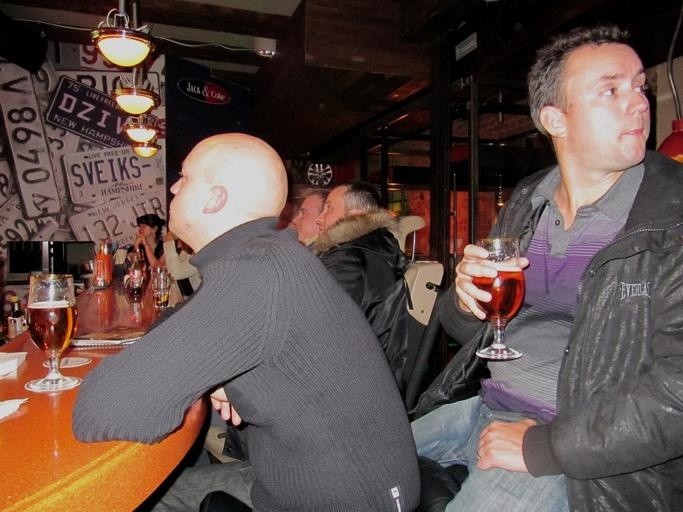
128;256;142;304
93;242;113;288
6;296;25;340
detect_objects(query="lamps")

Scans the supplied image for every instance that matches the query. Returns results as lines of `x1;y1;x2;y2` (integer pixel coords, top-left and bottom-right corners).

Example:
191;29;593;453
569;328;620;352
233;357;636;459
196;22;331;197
90;0;163;158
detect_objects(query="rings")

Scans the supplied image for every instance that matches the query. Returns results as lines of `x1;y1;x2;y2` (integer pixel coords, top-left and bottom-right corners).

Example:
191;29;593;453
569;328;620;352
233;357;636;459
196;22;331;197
475;446;482;459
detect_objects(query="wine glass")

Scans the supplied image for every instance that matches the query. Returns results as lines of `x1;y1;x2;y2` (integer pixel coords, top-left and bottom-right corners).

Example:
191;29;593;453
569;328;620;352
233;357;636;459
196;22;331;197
22;268;83;394
474;237;526;362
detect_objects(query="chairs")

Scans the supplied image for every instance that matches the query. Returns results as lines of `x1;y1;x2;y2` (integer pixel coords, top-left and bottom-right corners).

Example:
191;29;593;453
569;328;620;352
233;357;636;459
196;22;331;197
401;259;447;412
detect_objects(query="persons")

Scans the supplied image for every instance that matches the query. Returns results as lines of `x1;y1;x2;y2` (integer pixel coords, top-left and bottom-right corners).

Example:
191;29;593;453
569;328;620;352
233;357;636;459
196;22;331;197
70;131;424;511
122;179;410;463
406;23;680;511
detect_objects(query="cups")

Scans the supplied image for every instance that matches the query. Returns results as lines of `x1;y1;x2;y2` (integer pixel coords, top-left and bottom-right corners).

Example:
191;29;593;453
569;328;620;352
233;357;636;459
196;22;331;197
80;258;107;290
149;264;169;310
